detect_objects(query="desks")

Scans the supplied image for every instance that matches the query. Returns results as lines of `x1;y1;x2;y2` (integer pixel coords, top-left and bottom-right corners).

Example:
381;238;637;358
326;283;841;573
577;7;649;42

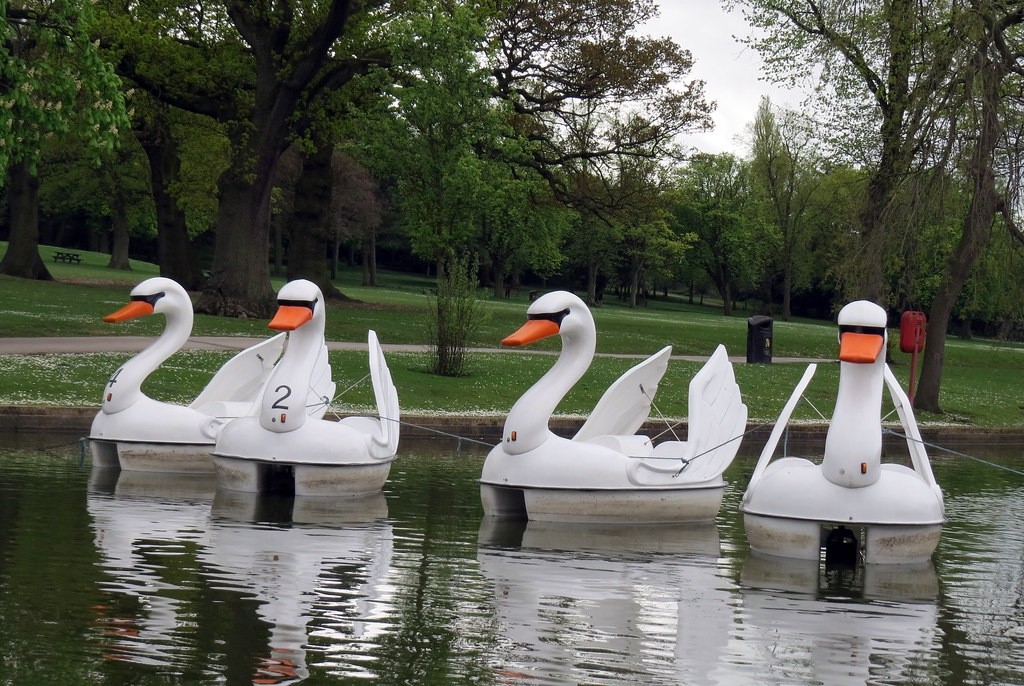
54;251;80;263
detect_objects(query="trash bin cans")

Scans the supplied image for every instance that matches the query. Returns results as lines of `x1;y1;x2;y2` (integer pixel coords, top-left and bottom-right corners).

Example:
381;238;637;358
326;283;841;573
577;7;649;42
529;290;537;301
747;316;774;363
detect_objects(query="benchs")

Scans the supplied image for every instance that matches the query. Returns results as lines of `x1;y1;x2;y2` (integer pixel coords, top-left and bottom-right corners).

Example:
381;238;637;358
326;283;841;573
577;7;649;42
52;255;81;264
